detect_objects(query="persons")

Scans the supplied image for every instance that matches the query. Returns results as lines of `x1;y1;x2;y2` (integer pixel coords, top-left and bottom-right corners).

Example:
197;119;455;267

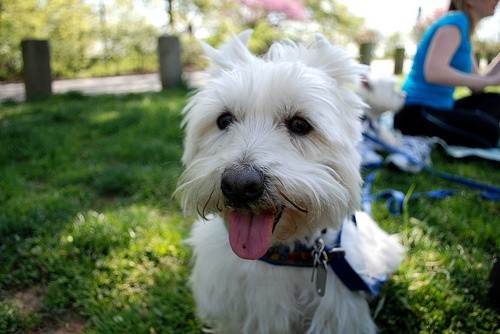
394;0;500;150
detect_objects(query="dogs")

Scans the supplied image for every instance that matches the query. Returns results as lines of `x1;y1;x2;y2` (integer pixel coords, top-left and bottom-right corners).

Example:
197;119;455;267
171;28;408;334
